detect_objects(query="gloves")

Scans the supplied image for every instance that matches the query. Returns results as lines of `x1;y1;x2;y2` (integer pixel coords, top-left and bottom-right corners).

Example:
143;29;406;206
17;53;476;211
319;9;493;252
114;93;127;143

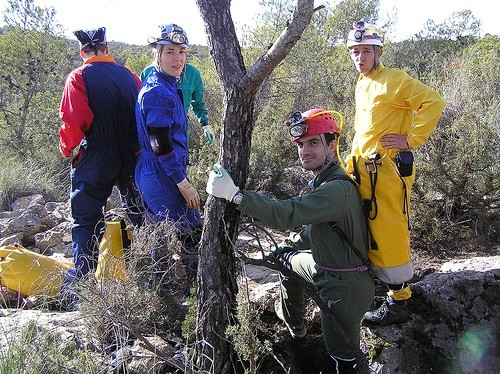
176;178;200;210
202;125;215;146
205;163;239;202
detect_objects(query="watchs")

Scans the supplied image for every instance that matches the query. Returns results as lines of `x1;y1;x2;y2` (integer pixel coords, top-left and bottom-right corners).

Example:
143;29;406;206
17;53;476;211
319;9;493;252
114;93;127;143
232;190;243;205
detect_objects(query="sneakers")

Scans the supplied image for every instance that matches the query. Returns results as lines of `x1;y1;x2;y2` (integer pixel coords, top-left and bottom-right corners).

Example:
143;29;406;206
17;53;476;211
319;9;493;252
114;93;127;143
274;297;306;338
361;300;411;328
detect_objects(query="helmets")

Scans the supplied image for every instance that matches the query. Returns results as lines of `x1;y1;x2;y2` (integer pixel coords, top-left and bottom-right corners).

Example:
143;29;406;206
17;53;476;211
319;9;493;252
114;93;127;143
146;24;189;48
73;26;109;50
346;20;384;48
286;109;341;142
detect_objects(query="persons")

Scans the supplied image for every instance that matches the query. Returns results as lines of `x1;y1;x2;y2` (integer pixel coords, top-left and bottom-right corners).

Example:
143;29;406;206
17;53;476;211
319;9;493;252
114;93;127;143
57;28;146;283
140;61;216;144
343;20;445;328
206;108;376;374
135;22;205;298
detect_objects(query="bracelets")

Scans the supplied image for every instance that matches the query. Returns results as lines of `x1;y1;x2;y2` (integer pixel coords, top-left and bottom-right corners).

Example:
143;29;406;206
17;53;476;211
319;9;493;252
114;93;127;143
178;182;191;190
406;136;410;149
